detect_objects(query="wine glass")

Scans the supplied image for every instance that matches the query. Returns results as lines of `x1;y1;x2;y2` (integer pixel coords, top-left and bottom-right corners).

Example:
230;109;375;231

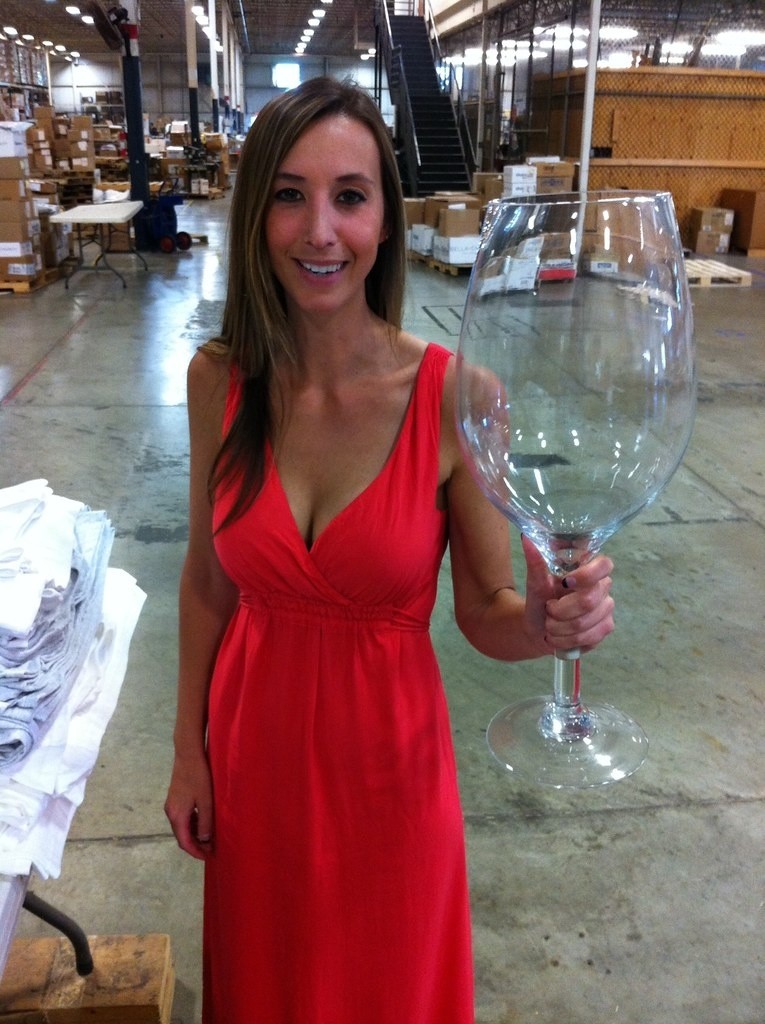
454;189;698;790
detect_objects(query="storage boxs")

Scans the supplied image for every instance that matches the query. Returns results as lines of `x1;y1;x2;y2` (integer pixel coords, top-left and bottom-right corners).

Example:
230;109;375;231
0;178;30;202
44;232;74;267
33;195;59;205
0;122;27;158
28;219;41;237
54;112;233;253
27;180;56;194
33;106;55;119
0;222;29;243
0;39;48;121
0;241;33;258
0;254;37;281
0;156;26;180
39;206;65;232
0;200;32;223
27;127;53;176
401;155;734;273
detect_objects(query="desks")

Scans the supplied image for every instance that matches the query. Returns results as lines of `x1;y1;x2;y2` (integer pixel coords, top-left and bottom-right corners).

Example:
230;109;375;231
48;201;149;291
0;567;148;979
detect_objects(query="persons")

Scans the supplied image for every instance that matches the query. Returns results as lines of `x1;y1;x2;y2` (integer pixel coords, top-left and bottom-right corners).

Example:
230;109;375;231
164;77;615;1024
